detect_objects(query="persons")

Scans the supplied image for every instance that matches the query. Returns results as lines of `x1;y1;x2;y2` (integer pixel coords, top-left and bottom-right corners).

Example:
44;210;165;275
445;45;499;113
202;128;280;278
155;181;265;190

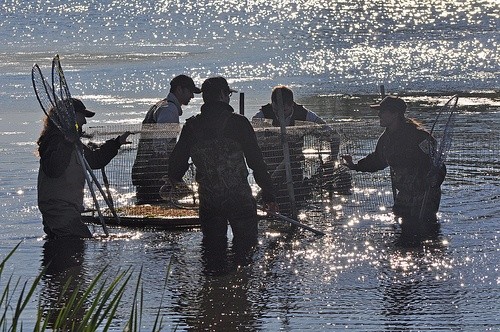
131;75;202;204
36;98;135;239
338;96;447;226
167;76;281;271
252;87;340;202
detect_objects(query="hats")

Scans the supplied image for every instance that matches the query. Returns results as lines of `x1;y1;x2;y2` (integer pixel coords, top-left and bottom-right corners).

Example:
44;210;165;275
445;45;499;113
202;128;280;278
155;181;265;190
170;74;201;94
370;96;407;115
64;97;96;118
201;77;238;95
